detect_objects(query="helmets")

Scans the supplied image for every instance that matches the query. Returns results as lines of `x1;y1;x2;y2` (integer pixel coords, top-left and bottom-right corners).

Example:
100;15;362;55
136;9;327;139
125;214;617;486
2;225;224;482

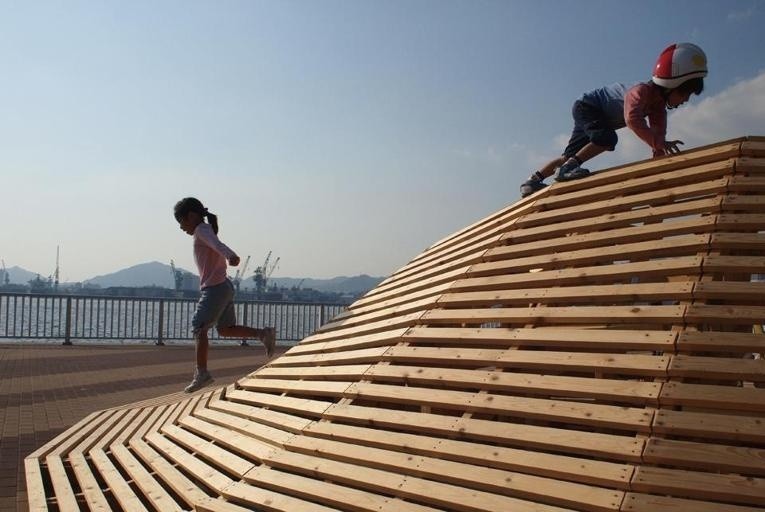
652;43;707;88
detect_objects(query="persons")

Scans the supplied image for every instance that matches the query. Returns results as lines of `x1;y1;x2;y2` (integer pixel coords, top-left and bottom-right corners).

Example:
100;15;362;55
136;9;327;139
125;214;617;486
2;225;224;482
520;42;708;199
174;197;276;394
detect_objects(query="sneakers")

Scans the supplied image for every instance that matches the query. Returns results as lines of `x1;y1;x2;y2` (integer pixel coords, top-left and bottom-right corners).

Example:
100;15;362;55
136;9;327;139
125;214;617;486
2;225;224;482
185;373;214;393
555;167;589;181
520;182;549;198
261;327;275;358
